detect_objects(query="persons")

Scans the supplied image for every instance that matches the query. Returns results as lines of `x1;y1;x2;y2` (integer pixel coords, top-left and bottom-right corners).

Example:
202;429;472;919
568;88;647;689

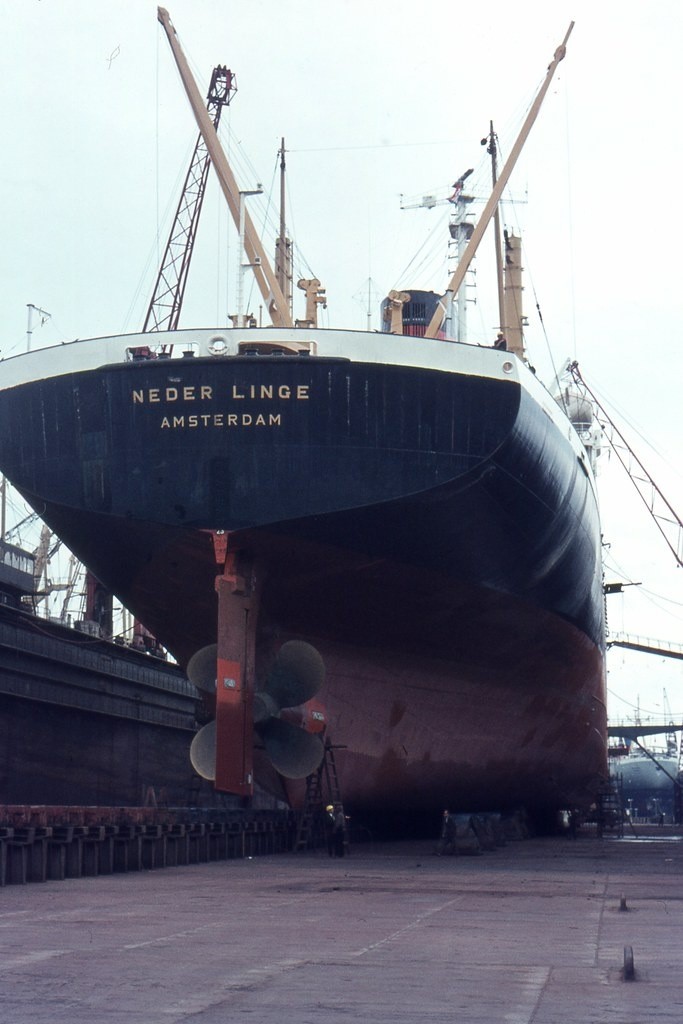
658;813;664;827
566;811;579;841
495;332;507;350
434;810;455;857
325;805;352;858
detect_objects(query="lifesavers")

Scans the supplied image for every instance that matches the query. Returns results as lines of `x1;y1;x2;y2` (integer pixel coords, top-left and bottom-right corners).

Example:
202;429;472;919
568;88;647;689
207;333;230;355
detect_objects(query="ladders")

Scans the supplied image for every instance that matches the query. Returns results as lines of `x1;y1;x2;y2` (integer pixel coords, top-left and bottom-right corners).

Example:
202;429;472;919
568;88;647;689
597;771;625;839
292;734;352;860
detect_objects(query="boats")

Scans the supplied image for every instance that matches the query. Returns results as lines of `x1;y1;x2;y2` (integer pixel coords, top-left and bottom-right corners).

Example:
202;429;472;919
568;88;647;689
0;0;683;837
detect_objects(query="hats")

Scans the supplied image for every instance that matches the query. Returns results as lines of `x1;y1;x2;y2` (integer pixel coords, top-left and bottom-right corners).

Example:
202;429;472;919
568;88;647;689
326;805;333;811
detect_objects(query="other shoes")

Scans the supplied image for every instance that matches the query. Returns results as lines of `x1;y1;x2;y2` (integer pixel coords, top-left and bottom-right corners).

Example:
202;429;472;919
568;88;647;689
434;851;440;856
